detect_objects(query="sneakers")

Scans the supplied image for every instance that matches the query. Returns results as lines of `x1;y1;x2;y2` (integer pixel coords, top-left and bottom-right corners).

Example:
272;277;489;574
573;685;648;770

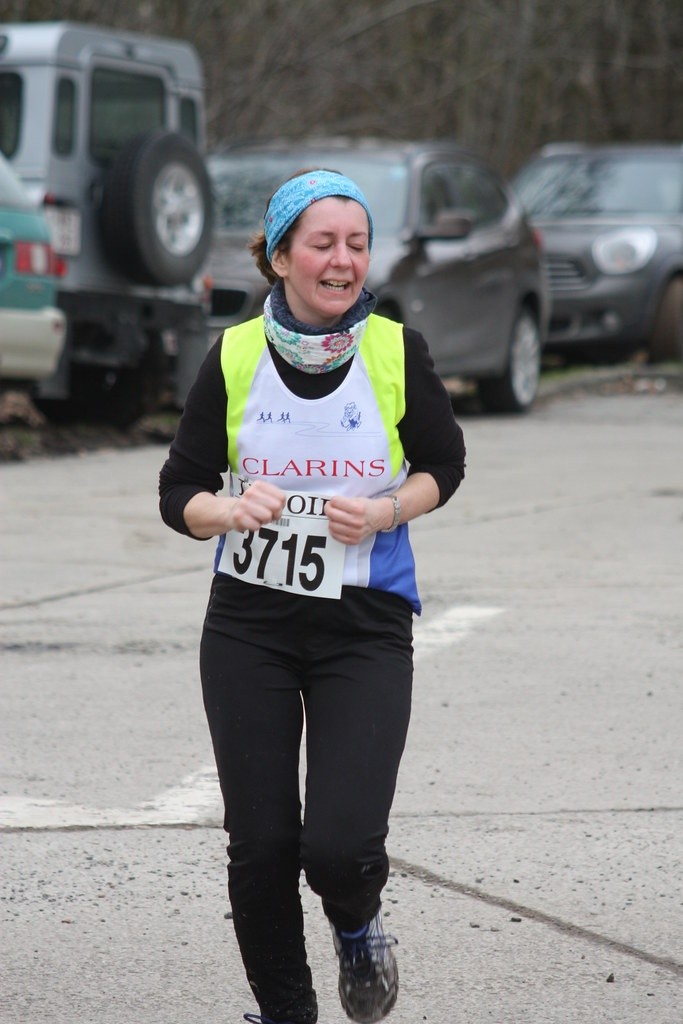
329;907;399;1024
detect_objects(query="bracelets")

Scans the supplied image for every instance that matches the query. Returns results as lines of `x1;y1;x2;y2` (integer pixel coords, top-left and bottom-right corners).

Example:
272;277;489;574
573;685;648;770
381;495;401;533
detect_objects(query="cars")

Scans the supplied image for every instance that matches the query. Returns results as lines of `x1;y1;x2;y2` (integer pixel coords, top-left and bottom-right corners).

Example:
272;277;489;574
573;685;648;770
507;136;683;372
0;147;69;386
159;135;555;415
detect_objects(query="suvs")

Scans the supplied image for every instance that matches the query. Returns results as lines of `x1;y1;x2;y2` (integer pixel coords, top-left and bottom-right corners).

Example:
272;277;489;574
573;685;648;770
1;23;222;427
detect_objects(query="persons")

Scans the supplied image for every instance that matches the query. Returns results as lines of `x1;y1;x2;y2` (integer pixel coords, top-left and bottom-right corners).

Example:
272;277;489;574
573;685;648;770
158;168;465;1024
